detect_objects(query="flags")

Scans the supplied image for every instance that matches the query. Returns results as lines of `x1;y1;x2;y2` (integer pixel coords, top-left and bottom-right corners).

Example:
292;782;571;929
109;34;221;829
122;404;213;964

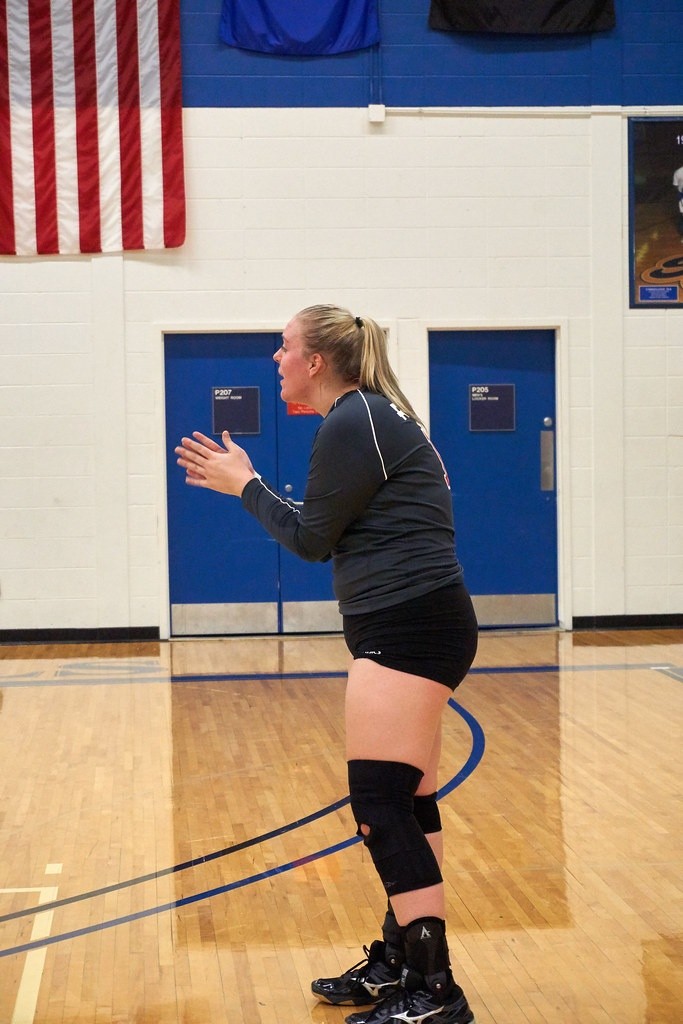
0;0;186;255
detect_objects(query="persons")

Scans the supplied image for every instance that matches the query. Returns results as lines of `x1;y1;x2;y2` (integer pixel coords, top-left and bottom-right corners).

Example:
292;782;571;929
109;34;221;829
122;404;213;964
174;303;478;1024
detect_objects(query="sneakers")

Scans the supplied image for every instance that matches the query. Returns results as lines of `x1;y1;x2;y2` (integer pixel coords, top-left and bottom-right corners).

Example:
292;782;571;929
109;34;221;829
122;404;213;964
311;940;403;1006
344;969;474;1024
